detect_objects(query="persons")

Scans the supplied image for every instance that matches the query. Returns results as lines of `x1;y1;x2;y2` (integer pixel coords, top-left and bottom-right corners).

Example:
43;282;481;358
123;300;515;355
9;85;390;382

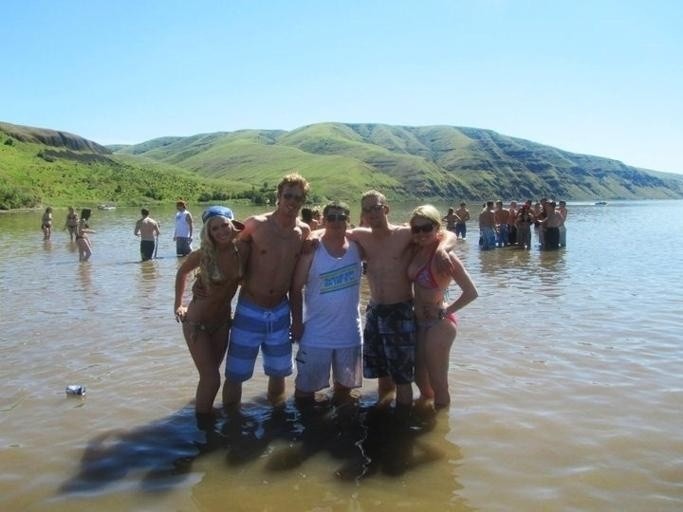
358;203;479;406
75;206;97;261
478;197;568;251
306;188;458;406
172;200;194;257
441;206;462;234
288;197;365;400
221;172;310;406
41;207;54;236
63;205;79;239
134;207;161;261
297;203;357;230
174;205;248;414
454;203;470;237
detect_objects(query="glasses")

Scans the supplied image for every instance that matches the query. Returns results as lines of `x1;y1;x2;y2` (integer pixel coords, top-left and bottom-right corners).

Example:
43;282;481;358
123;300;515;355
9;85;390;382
284;193;302;203
326;215;345;222
411;222;434;234
362;205;384;212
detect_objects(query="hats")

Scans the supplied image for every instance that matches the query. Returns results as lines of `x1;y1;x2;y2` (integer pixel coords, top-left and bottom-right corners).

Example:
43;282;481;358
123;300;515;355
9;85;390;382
202;206;245;230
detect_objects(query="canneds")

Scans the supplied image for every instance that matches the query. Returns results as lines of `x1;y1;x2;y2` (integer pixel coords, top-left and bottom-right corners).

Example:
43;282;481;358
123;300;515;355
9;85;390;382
65;385;86;396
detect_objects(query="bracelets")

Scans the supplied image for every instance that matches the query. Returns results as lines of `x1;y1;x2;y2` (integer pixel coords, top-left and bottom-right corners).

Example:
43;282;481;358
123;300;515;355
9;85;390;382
438;306;448;321
